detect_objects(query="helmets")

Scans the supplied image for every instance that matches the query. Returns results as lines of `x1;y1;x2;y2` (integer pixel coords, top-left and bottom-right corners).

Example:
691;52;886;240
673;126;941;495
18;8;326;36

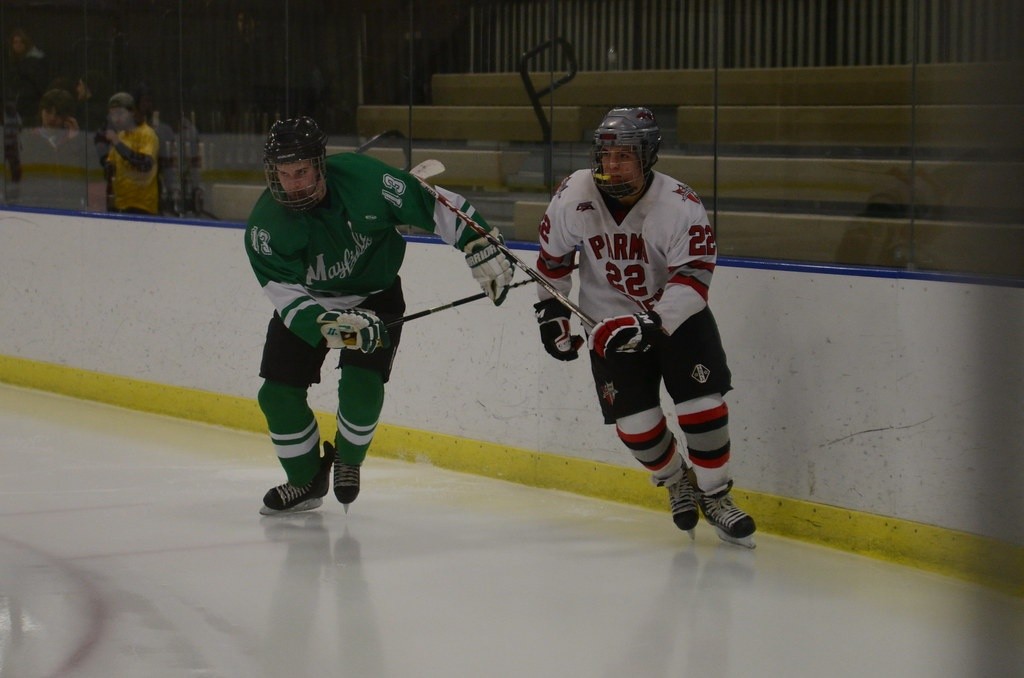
591;105;665;200
261;115;329;214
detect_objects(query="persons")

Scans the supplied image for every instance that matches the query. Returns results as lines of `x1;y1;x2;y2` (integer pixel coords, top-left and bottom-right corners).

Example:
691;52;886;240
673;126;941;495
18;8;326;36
8;30;49;124
617;550;737;678
537;107;758;549
18;90;81;207
260;511;386;678
245;116;514;517
99;94;158;216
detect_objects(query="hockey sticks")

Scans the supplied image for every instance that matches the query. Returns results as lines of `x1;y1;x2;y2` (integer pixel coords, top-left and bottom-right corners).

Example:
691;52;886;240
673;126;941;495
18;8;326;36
409;159;598;329
385;262;580;329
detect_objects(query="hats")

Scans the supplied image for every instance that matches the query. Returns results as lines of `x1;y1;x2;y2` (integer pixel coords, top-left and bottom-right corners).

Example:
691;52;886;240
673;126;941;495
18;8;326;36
107;91;136;109
39;87;74;108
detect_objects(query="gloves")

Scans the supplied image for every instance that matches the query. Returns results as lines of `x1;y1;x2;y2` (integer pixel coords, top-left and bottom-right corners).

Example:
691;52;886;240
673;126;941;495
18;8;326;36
587;310;663;363
317;307;391;354
464;226;515;306
534;297;584;362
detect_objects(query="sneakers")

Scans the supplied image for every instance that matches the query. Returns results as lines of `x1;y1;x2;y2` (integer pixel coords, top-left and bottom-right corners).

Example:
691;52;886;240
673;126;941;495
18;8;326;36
695;478;757;550
653;452;699;540
260;441;335;515
334;446;360;504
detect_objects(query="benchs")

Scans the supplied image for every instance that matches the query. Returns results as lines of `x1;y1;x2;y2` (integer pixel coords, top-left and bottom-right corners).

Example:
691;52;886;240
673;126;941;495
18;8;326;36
190;61;1023;279
